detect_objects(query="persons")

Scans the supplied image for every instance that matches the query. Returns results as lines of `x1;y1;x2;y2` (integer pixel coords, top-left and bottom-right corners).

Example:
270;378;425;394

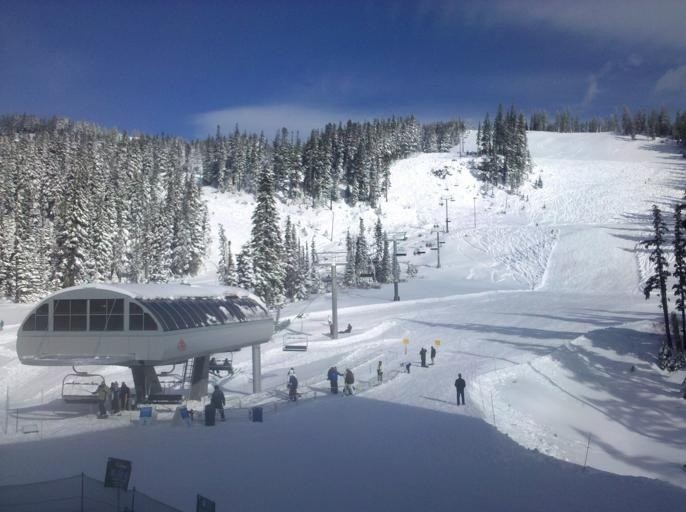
376;360;384;382
286;374;297;403
344;368;355;395
329;366;344;394
455;373;466;405
288;368;295;377
222;358;234;375
419;347;428;366
210;357;220;374
431;346;436;365
89;380;131;415
210;385;226;421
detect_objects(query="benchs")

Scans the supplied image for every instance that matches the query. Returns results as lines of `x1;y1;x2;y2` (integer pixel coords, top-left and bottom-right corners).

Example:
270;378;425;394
209;365;232;370
282;346;307;351
63;395;98;403
144;394;185;404
